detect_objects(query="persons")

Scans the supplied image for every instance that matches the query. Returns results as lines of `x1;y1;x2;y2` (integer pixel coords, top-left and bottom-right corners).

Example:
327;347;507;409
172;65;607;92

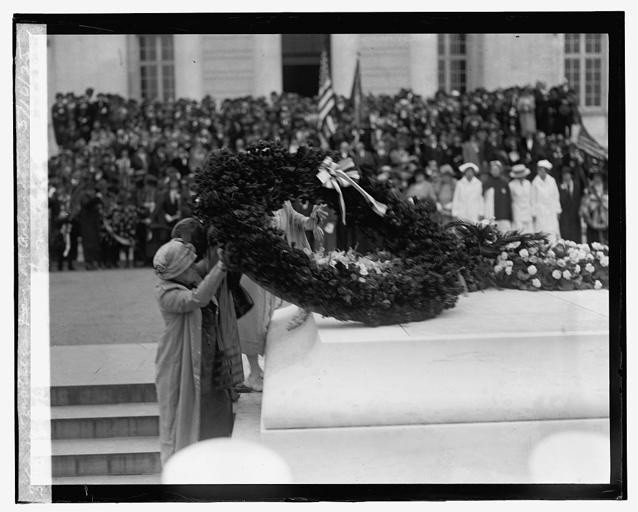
48;82;608;272
153;238;254;469
237;200;329;392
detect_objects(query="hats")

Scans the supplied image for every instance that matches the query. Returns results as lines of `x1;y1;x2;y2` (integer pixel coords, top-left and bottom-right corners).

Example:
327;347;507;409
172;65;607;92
153;218;206;280
537;159;553;170
509;164;531;178
459;163;479;173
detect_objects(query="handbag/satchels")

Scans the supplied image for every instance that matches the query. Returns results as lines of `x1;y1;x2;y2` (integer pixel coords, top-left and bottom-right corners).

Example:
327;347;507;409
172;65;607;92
230;284;254;319
